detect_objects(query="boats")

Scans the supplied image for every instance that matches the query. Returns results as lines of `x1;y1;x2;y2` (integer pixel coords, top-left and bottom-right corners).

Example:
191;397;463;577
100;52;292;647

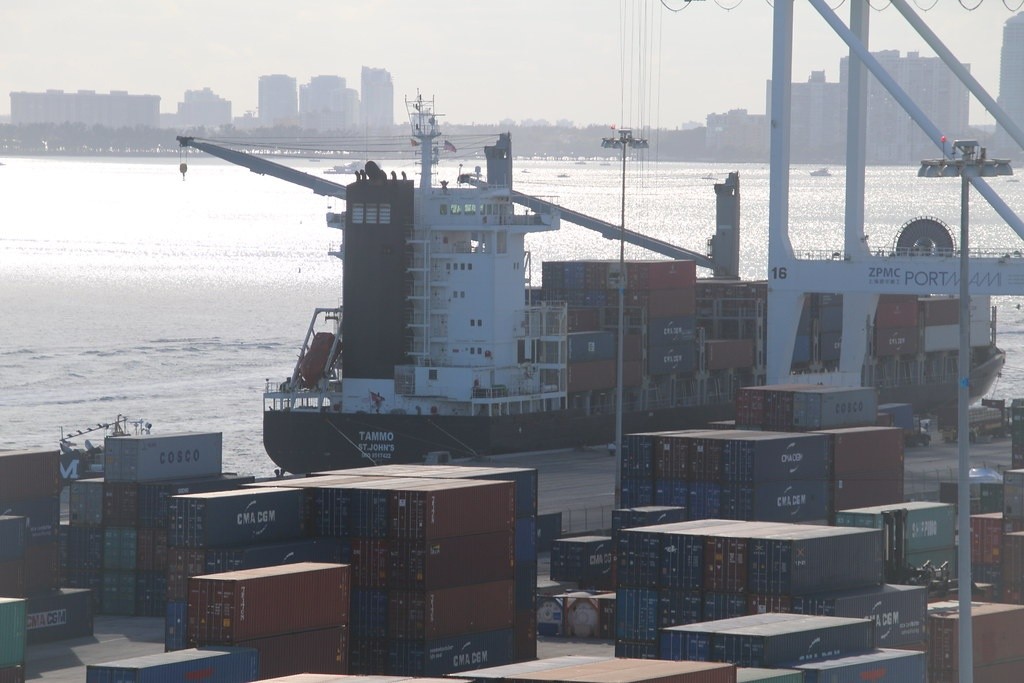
263;86;1005;472
323;159;379;174
810;169;830;176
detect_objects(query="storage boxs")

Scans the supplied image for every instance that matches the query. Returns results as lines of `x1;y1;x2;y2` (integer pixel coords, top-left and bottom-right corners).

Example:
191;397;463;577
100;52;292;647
103;431;222;482
0;448;61;502
26;587;95;646
24;541;60;587
568;360;615;393
537;382;1024;683
525;288;541;304
0;596;28;669
0;501;60;545
541;258;990;376
57;464;537;683
616;359;642;388
0;559;29;598
0;515;25;561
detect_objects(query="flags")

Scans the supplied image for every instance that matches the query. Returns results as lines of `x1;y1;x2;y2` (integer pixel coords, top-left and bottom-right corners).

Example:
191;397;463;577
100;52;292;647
444;140;456;152
411;139;419;146
